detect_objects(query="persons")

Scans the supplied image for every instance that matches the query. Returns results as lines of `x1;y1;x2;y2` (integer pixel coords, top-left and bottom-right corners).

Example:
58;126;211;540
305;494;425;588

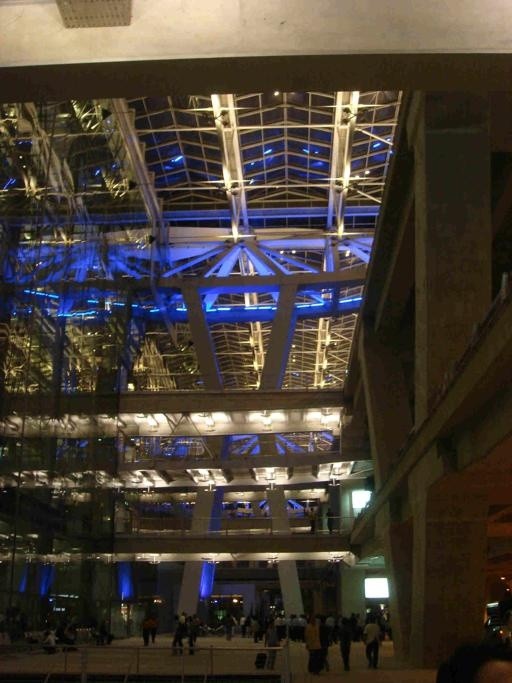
171;607;392;674
308;507;319;534
436;591;512;683
0;608;108;655
142;617;157;647
325;507;336;535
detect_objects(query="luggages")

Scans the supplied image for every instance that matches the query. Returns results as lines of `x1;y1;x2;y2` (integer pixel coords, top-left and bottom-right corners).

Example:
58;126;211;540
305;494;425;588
255;652;267;670
